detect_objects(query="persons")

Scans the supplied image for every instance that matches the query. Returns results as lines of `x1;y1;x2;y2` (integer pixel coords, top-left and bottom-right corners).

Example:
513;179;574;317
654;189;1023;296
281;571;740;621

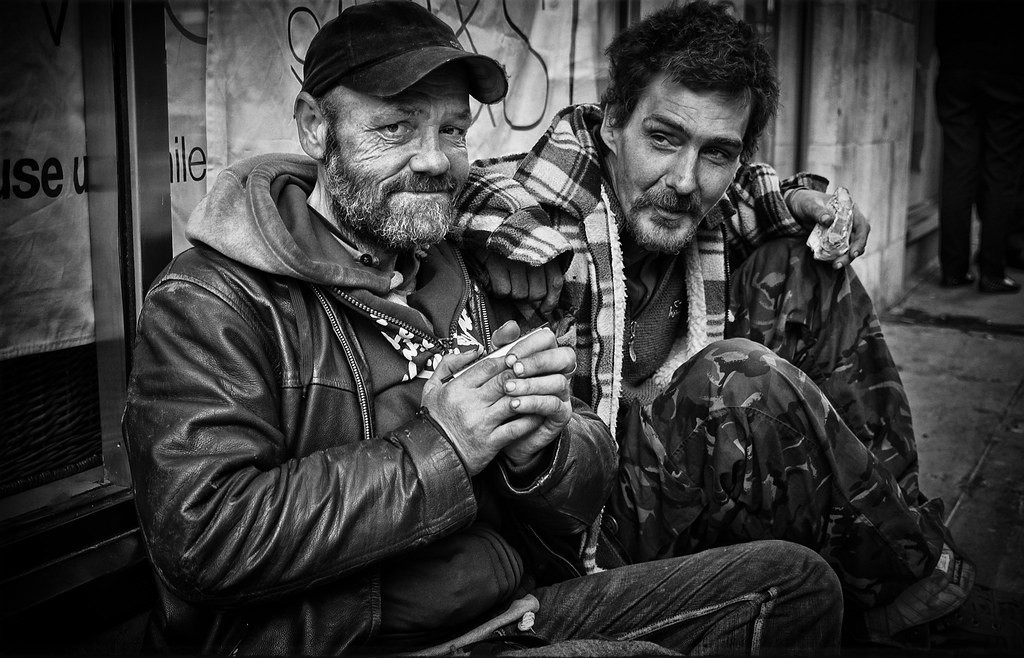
121;1;844;657
934;0;1024;296
450;4;1024;658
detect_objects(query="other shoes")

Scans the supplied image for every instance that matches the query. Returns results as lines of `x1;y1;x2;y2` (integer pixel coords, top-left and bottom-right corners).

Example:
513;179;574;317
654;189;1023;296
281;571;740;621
941;273;975;288
979;272;1019;293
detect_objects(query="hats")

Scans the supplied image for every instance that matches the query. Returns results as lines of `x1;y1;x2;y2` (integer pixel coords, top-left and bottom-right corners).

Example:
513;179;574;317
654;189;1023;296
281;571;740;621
302;0;510;105
